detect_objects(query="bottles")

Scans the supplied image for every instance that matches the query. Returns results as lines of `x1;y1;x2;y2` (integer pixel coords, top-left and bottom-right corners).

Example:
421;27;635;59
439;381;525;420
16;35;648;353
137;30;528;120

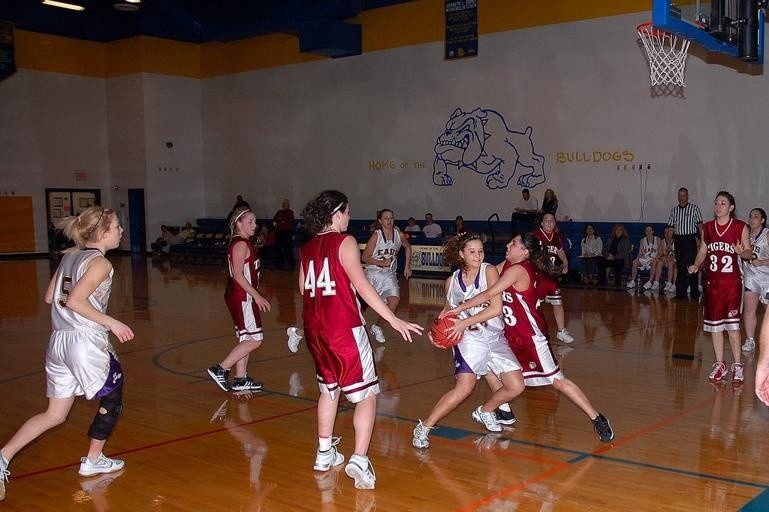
636;274;641;293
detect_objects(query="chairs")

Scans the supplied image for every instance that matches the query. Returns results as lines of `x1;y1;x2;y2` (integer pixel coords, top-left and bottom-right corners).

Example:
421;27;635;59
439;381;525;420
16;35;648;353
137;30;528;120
358;229;374;243
559;221;670;286
154;226;230;266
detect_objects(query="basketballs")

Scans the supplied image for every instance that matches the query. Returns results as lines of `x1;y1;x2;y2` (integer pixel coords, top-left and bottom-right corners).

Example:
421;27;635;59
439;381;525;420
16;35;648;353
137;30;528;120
432;313;463;348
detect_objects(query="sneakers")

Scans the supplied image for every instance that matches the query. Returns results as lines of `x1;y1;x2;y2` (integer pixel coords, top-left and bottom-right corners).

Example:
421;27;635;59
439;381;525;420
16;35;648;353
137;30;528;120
474;432;510;453
207;365;263;392
287;327;303;353
625;280;676;292
556;329;574;344
0;453;10;501
709;338;755;382
472;406;517;431
314;447;345;471
710;381;744;390
208;391;261;425
78;451;125;476
289;372;305;397
80;468;125;492
553;347;574;355
373;346;386;363
370;324;385;343
591;414;614;441
627;288;676;297
344;455;376;489
413;423;431;450
315;471;376;512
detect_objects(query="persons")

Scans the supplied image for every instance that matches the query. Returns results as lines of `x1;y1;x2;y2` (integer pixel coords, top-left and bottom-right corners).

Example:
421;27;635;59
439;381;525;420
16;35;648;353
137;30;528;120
688;191;752;384
525;212;573;342
362;209;414;344
423;213;442;238
252;226;275;263
0;205;136;498
410;384;618;512
451;216;464;234
596;225;630;289
286;373;321;402
273;199;296;271
286;325;305;352
310;462;375;512
581;224;603;284
370;345;407;473
516;188;538;231
554;343;576;372
404;217;420;238
740;207;768;351
538;189;559;224
577;288;703;411
209;388;277;512
666;189;700;300
445;234;614;441
627;226;660;289
154;223;195;255
411;231;525;447
78;466;127;511
696;379;746;512
206;209;270;391
298;190;426;489
754;288;769;407
652;228;676;291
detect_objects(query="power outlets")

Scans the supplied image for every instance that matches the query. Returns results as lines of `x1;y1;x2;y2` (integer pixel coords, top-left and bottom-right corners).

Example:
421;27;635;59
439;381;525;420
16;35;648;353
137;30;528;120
638;163;643;170
647;163;653;170
